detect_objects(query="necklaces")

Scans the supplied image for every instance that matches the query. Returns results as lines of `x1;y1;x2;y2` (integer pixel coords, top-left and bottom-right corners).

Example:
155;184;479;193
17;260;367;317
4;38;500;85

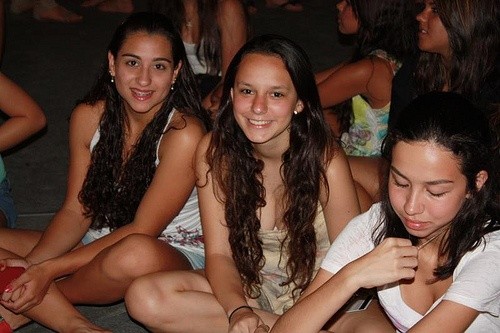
186;17;194;26
418;230;444;250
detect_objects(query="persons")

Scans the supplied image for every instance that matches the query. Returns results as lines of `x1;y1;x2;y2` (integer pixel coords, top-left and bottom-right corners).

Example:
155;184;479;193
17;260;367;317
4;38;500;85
0;15;205;333
268;91;500;333
124;34;397;333
0;0;500;228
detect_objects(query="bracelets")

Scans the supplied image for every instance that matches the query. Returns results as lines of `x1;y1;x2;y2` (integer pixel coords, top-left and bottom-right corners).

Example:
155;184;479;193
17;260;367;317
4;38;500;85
228;305;253;323
